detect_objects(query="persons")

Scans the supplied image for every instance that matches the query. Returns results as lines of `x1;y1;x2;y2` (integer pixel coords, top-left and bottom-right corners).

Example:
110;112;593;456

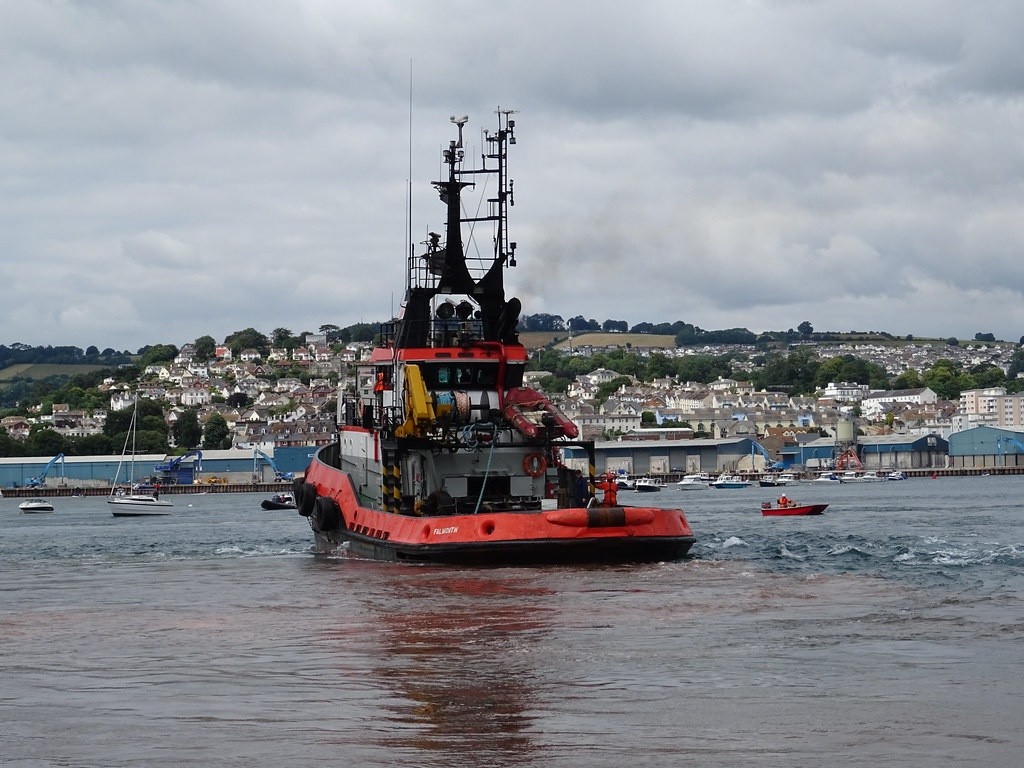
556;463;589;507
280;494;286;503
272;490;280;504
594;473;618;510
153;481;162;501
777;493;791;508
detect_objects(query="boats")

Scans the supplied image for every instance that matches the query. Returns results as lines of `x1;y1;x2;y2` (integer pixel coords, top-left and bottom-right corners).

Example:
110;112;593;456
653;478;667;488
712;475;752;488
290;57;698;567
801;469;908;485
677;475;709;491
696;471;718;485
108;392;174;517
776;474;799;485
634;477;662;492
761;500;830;516
19;499;54;514
758;475;786;487
71;493;87;498
613;474;637;489
261;495;298;510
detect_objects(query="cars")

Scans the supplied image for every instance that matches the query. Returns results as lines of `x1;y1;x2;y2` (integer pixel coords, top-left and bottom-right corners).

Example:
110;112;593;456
670;467;686;473
206;476;225;484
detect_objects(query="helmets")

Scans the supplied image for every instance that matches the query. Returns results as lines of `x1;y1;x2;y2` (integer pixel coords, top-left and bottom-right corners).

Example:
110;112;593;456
606;473;614;480
782;493;786;498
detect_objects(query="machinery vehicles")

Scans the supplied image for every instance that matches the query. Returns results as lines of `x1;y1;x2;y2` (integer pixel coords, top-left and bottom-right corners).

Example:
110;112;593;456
252;448;293;484
24;453;67;489
996;435;1024;466
153;450;203;485
751;441;785;473
831;448;864;471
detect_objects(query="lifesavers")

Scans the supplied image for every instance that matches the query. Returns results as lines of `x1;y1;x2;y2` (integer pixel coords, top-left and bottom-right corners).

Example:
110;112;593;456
524;454;546;476
358;399;364;416
